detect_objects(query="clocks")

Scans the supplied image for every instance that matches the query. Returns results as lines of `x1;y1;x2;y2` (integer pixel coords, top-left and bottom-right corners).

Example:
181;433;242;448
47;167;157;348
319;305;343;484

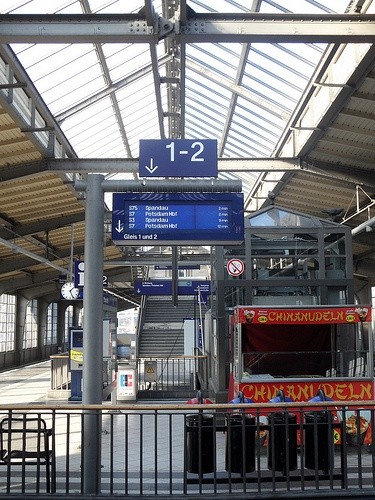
61;282;79;300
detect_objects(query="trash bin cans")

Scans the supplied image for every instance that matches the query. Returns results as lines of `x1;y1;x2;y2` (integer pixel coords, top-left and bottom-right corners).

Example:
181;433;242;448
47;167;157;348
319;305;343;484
113;357;139;404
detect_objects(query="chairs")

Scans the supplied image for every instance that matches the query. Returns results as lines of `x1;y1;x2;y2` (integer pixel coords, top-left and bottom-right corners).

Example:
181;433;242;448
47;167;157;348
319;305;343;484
0;418;57;496
